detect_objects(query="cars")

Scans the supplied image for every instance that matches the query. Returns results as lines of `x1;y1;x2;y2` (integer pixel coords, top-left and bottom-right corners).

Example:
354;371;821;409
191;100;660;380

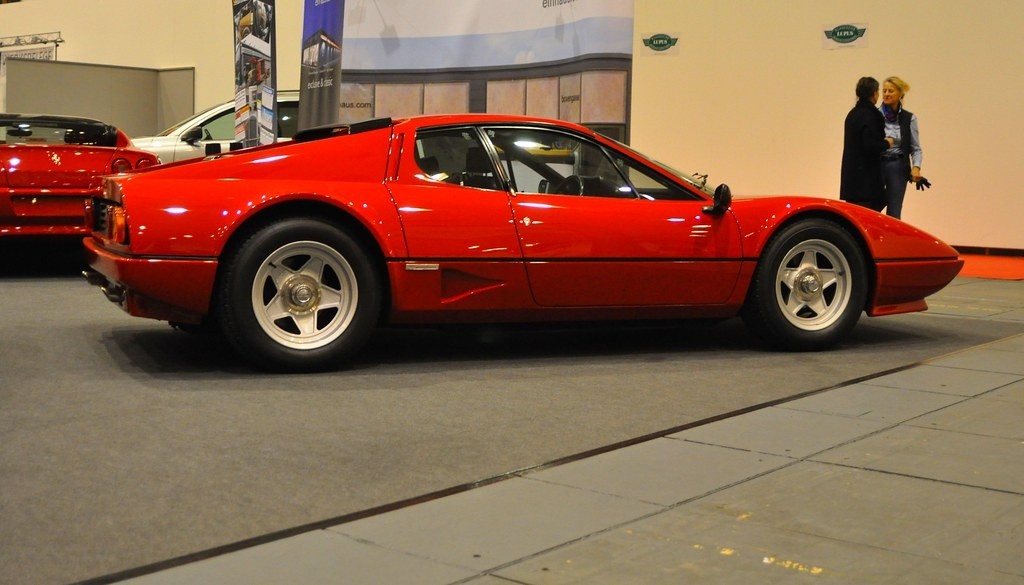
0;110;162;272
84;114;963;373
129;88;300;165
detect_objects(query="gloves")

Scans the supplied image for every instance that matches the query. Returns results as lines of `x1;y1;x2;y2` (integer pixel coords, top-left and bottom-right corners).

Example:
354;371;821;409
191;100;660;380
909;174;932;191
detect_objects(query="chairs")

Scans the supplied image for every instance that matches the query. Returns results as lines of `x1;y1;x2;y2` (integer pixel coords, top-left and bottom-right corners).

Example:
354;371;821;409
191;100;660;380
464;146;502;191
63;122;97;147
418;156;461;185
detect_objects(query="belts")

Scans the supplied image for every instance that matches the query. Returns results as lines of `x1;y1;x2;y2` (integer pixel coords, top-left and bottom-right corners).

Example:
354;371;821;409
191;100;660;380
879;153;904;160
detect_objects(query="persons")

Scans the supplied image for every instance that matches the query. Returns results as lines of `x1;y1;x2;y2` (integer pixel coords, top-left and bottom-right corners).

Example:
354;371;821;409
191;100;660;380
840;76;893;213
878;77;922;220
